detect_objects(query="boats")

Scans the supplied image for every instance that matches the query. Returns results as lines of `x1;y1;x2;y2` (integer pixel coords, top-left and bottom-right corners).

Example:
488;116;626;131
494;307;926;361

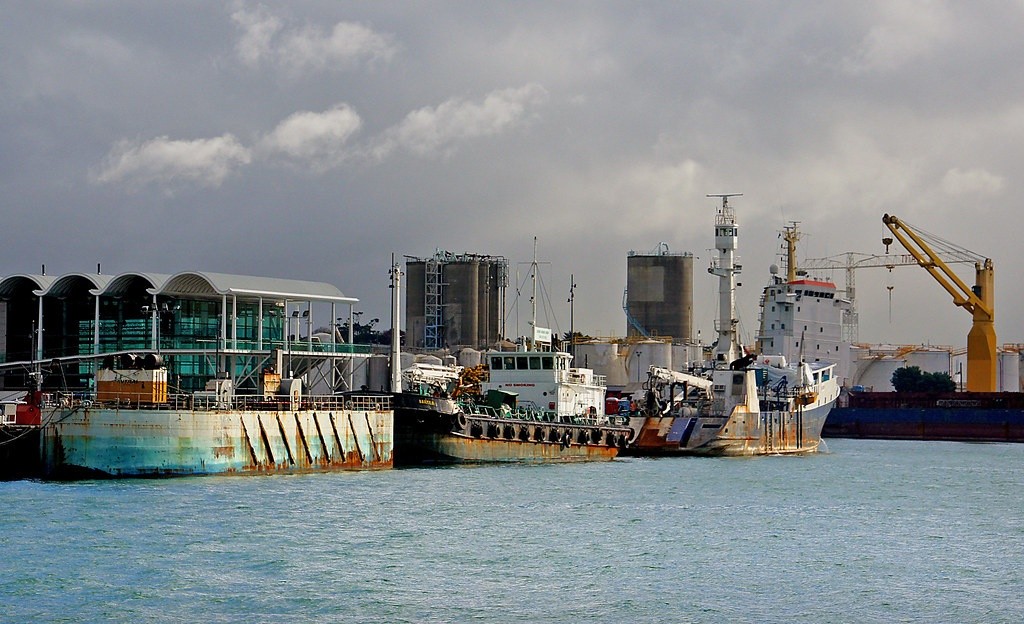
401;336;637;465
36;348;393;478
606;319;844;461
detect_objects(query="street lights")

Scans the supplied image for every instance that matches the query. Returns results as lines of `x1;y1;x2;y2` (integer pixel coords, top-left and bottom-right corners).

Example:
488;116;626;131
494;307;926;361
566;274;577;367
387;253;395;364
270;308;309;377
140;302;181;359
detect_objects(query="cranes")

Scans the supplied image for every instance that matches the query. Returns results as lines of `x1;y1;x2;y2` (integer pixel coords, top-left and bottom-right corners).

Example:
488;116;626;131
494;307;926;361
881;212;999;393
794;250;985;349
